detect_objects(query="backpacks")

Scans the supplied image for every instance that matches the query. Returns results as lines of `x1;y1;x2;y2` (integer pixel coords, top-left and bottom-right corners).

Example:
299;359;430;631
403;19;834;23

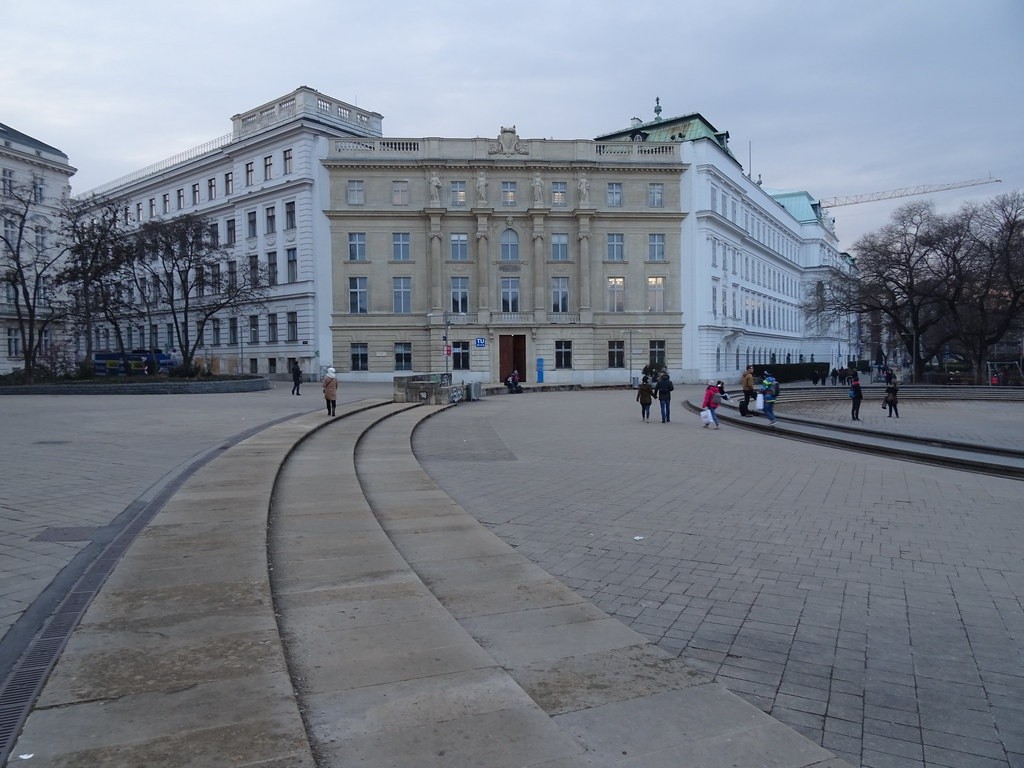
713;392;722;404
849;388;856;398
739;401;747;416
762;376;780;397
516;386;523;393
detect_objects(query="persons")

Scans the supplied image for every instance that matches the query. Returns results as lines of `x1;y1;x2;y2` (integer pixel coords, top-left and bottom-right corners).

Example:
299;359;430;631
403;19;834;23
849;377;862;422
291;361;302;396
702;380;721;430
883;382;900;419
653;373;675;423
878;365;900;392
636;375;656;423
509;370;522;394
757;369;780;426
321;367;338;417
811;367;860;387
861;365;873;375
742;364;758;417
504;374;517;394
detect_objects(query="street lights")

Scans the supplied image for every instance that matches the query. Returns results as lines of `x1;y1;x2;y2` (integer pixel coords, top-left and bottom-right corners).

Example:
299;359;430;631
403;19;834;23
900;330;915;384
621;329;643;383
427;310;466;373
240;325;257;374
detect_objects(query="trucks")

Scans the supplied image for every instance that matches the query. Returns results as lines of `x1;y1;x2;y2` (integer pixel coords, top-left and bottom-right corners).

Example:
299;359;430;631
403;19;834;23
92;347;183;380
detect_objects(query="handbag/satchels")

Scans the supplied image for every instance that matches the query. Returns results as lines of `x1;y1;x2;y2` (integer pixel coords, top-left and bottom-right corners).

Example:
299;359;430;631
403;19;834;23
891;378;898;383
700;409;713;424
298;373;303;384
755;393;764;410
323;389;325;393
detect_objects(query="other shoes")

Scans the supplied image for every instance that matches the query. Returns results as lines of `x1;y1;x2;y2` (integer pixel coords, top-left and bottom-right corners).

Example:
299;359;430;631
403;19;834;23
667;419;670;422
292;390;294;394
646;418;649;423
661;420;665;423
296;393;301;395
746;414;753;417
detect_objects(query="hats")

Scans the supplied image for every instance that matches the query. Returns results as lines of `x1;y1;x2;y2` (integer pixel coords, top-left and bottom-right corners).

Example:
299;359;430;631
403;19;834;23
514;370;518;373
706;380;716;385
327;368;336;373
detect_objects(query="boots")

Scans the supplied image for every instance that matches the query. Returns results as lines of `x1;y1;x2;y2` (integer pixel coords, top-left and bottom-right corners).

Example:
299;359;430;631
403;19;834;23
328;408;331;415
332;407;335;416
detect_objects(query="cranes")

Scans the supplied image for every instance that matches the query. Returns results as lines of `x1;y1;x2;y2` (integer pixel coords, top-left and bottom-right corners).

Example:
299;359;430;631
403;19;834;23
819;173;1002;210
